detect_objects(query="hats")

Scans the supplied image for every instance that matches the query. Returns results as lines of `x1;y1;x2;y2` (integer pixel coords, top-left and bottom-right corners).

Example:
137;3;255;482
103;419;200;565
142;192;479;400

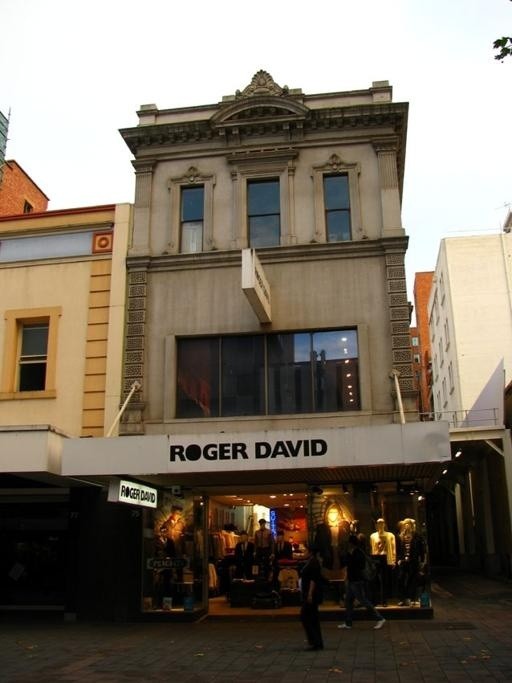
240;530;246;535
258;519;266;524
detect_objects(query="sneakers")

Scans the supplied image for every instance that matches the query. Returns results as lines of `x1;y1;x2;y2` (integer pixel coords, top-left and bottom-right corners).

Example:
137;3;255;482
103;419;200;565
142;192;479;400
371;617;387;630
302;641;324;652
335;621;354;631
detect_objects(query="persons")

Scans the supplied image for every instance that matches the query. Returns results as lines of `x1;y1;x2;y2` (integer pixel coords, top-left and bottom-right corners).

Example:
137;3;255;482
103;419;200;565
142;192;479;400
152;504;187;610
254;518;273;559
397;518;425;606
350;518;367;550
273;530;293;561
368;519;396;607
336;535;387;629
234;531;254;562
298;541;326;652
